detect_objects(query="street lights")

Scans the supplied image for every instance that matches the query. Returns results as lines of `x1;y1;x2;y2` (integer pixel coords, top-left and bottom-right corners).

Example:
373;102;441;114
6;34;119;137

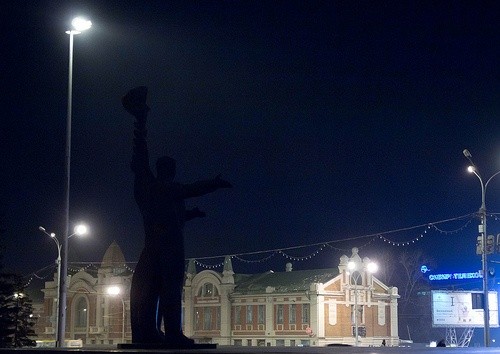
53;14;94;346
108;286;127;342
462;149;500;346
40;223;88;347
345;260;380;346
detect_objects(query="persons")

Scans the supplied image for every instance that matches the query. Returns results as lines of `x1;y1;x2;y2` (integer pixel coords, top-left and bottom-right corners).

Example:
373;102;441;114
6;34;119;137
128;98;235;350
